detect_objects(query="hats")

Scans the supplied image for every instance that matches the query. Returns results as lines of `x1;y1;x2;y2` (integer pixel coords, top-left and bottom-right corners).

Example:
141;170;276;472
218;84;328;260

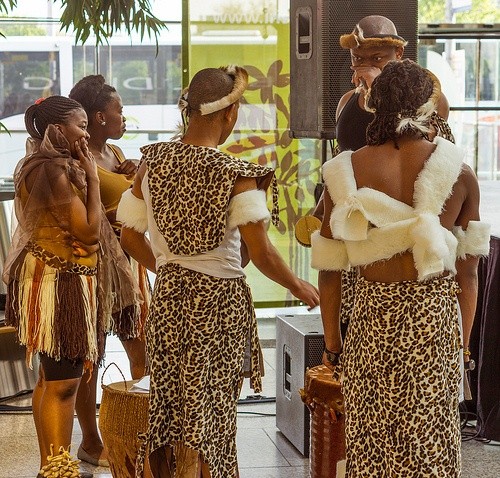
339;15;408;50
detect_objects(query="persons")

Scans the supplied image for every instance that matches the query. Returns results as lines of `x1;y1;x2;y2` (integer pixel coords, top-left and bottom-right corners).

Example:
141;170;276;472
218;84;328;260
317;59;492;478
5;97;101;478
311;14;446;224
119;65;321;478
67;75;153;469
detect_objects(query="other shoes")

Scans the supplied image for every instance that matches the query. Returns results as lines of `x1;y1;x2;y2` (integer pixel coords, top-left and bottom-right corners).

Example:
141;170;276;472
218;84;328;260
77;444;110;467
36;443;82;478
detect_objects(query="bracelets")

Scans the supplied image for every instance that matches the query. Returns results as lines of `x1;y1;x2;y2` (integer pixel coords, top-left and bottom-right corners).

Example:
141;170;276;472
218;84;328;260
325;347;344;362
462;348;471;356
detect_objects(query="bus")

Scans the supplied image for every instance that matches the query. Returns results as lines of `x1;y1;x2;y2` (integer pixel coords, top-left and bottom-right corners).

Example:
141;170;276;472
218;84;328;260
0;25;500;318
